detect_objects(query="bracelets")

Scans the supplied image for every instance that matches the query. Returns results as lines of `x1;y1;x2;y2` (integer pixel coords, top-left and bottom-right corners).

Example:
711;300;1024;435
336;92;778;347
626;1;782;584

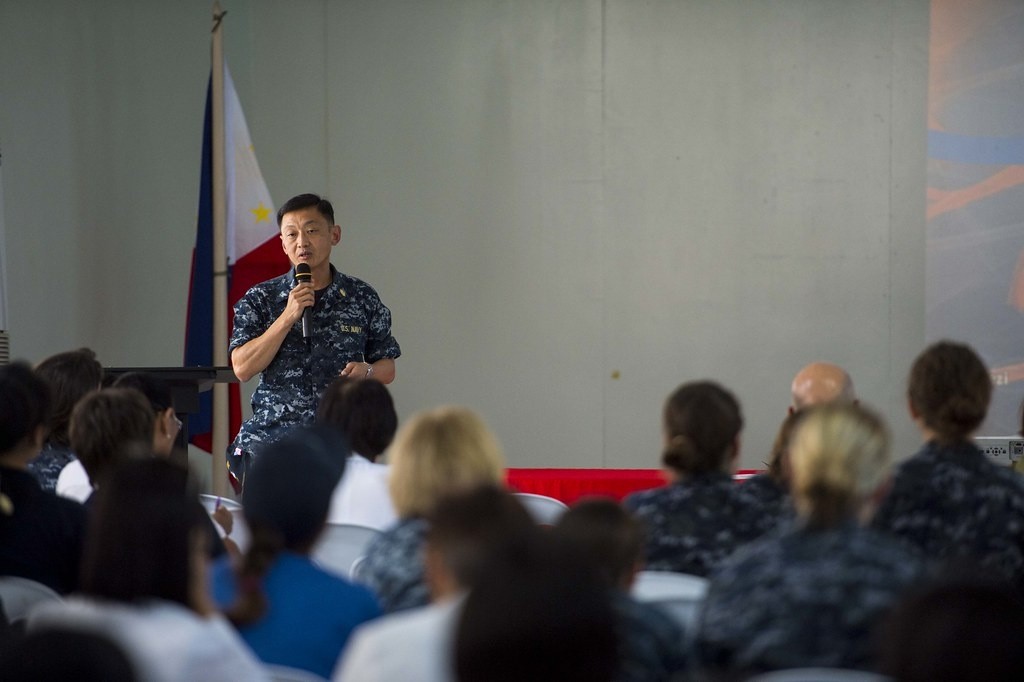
365;362;375;381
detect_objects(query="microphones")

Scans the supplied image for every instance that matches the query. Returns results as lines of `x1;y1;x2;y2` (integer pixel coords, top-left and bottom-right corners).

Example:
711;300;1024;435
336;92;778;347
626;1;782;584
296;263;312;337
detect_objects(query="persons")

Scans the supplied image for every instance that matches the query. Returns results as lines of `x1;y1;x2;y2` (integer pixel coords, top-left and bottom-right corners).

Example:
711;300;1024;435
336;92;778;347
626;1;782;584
1;337;1023;682
230;194;404;483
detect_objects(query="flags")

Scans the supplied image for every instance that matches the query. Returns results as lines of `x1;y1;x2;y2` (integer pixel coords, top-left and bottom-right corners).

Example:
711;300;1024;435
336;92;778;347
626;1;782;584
176;61;292;459
